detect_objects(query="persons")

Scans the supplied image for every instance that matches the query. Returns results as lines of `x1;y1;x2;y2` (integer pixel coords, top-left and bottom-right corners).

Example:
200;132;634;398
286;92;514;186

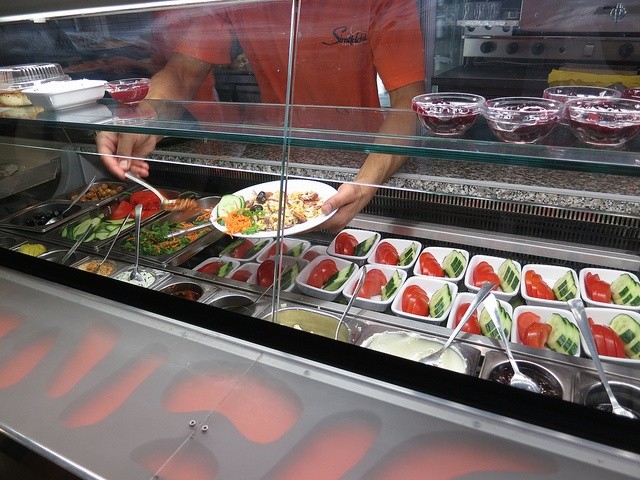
94;35;223;132
95;0;426;236
237;47;254;75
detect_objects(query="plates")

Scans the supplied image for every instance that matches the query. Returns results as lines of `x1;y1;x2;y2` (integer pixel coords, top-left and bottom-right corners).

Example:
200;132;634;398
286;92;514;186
191;257;241;279
367;238;422;273
245;254;311;293
580;306;639;369
227;262;261;282
300;245;329;263
62;68;99;74
391;275;459;326
510;305;583;358
326;228;381;267
341;264;408;313
463;254;522;303
579;267;640;312
20;79;109;111
520;263;581;310
83;45;133;49
446;292;513;342
294;254;360;301
219;237;274;266
413;246;469;284
210;179;340;239
255;237;312;263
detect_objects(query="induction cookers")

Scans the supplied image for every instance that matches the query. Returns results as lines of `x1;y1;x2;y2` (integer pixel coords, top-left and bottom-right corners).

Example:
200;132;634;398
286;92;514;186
431;62;640;90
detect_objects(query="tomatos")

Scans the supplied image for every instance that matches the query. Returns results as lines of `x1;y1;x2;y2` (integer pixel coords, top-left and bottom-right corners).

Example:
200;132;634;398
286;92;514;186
456;304;481;335
230;240;253;259
352;269;387;299
196;261;224;277
584;271;612;303
420;253;446;278
268;241;287;256
518;312;553;349
303;250;319;261
473;261;499;291
402;285;429;316
307;258;335;288
525;269;555;299
105;189;168;219
257;260;284;287
231;270;252;282
374;242;398;265
587;318;626;358
334;233;358;255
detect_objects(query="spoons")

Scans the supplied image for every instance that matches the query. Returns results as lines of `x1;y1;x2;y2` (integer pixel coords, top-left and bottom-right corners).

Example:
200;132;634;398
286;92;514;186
483;291;544;394
125;170;198;214
93;213;131;275
129;205;147;283
420;281;495;369
44;175;98;227
568;298;640;421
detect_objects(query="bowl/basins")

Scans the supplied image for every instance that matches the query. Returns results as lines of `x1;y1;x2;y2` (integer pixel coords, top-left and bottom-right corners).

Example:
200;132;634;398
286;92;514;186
567;97;640;146
480;96;566;145
103;77;152;106
411;91;487;138
542;86;622;131
623;88;640;101
